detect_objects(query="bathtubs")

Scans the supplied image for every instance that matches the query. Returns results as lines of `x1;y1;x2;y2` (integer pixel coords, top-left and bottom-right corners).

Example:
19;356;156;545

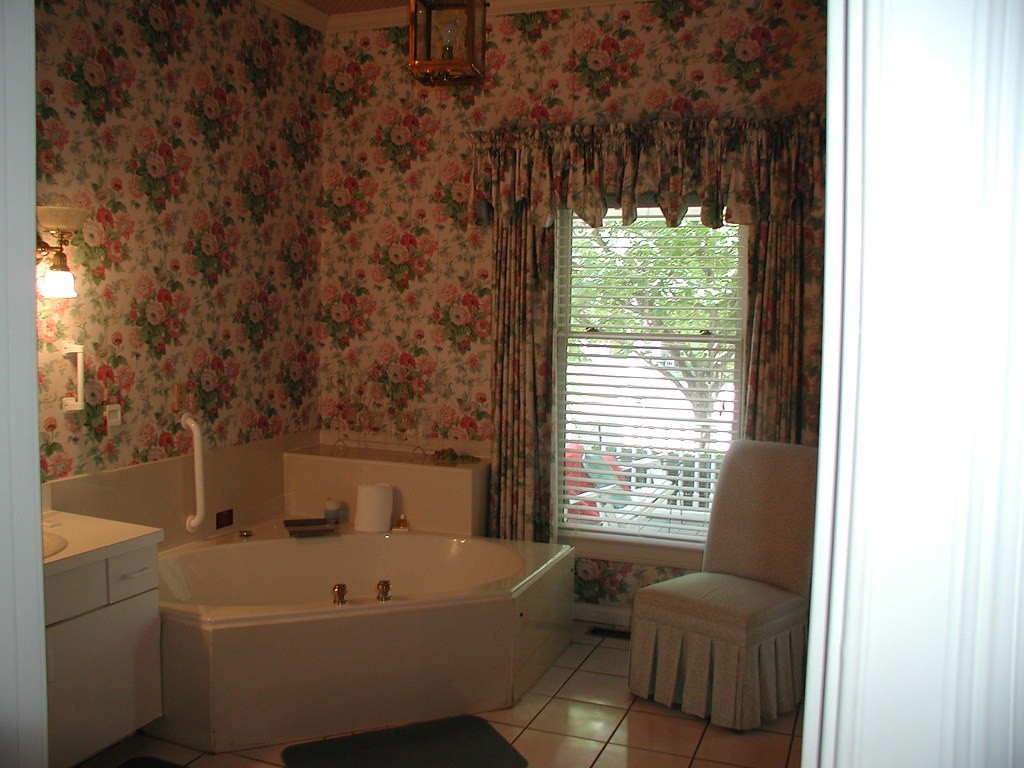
138;514;576;754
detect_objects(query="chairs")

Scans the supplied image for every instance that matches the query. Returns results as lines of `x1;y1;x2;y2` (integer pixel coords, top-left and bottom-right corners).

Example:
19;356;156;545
628;440;818;731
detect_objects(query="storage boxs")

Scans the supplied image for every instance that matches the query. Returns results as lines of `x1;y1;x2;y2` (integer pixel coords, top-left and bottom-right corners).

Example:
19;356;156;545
145;532;575;753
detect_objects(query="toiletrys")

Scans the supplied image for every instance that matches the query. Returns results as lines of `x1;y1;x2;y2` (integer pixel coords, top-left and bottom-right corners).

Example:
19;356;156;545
397;514;407;529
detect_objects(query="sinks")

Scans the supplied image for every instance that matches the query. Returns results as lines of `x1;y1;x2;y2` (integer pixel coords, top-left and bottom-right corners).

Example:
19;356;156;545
42;530;68;559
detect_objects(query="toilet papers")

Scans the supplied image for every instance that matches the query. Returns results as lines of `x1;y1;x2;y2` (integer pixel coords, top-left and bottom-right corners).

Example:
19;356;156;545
353;482;394;533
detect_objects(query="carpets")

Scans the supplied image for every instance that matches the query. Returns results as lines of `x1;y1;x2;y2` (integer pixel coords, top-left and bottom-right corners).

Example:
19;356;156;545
280;714;528;768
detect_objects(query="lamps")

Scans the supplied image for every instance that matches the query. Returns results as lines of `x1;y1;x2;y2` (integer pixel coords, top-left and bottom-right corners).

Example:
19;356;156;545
407;0;491;86
36;206;86;299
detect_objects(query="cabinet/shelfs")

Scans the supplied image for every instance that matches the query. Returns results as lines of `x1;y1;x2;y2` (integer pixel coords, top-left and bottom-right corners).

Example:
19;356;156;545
43;528;165;768
